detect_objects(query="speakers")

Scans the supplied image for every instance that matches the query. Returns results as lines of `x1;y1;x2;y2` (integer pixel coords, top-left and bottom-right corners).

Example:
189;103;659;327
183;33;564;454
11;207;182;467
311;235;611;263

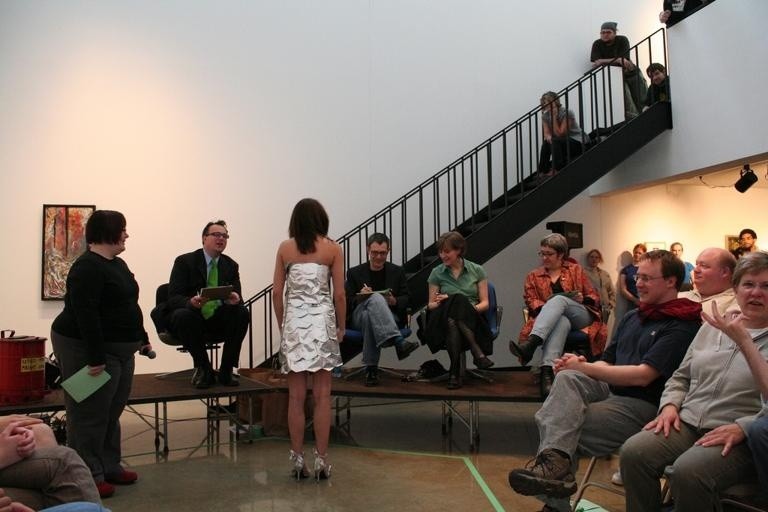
546;220;585;251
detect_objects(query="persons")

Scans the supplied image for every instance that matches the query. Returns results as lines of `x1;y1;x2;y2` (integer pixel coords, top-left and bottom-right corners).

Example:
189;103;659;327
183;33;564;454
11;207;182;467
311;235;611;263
535;92;588;180
641;63;670;113
167;220;250;389
51;210;151;496
733;229;759;260
659;0;703;27
0;490;111;511
0;414;57;447
619;244;647;311
678;248;742;317
620;251;768;512
701;301;764;453
272;199;345;483
509;233;609;398
591;21;648;112
427;231;495;390
670;242;697;291
584;250;616;325
345;233;420;387
509;250;702;512
0;422;101;505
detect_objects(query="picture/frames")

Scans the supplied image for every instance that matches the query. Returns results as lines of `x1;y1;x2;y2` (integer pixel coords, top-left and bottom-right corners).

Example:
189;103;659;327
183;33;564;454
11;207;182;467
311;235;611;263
644;241;666;252
41;205;97;301
725;234;739;252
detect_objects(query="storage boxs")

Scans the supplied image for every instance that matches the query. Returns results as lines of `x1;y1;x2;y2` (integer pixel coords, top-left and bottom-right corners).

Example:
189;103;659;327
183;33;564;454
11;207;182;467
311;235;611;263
0;329;47;405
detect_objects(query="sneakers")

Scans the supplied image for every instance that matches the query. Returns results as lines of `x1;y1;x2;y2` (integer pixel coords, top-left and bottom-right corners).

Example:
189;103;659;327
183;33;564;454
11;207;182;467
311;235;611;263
95;480;114;498
106;467;138;486
509;449;578;500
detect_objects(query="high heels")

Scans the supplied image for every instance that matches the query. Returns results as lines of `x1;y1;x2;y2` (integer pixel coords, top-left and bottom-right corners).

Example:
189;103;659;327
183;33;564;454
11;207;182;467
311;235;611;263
288;448;306;483
312;446;332;482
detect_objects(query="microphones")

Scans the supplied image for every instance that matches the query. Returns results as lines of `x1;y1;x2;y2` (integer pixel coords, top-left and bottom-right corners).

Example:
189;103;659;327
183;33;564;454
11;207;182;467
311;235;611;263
139;345;157;360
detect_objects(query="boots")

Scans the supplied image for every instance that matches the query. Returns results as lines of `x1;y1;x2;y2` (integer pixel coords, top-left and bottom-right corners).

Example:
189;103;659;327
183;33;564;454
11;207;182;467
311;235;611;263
540;365;555;397
508;336;541;366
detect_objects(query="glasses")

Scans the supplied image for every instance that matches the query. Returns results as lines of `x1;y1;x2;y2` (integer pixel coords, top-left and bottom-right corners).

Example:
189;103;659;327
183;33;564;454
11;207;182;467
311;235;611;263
632;273;669;283
368;249;389;258
738;279;768;295
206;232;229;240
537;249;559;258
600;31;615;35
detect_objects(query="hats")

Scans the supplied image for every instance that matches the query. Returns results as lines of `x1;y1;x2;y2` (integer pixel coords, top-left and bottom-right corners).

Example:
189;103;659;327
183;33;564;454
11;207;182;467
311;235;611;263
601;22;617;31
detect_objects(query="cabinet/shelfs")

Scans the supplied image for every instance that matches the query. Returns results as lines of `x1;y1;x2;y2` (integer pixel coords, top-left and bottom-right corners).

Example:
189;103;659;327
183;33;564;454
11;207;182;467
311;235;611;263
274;365;543;449
1;367;271;455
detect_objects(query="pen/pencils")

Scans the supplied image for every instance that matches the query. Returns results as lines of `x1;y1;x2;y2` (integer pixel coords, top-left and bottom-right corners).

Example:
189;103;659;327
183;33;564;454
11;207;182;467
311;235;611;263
196;291;201;305
364;282;367;287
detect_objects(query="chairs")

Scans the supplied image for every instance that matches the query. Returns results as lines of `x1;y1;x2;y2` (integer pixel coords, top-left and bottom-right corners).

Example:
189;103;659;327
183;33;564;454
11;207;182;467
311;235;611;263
149;282;240;381
420;281;501;383
336;288;413;382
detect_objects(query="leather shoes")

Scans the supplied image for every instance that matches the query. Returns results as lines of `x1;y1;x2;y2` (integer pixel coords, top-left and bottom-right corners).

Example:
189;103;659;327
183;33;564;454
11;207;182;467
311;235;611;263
191;367;215;388
217;372;240;387
473;356;494;370
447;374;462;389
364;372;380;387
395;342;419;361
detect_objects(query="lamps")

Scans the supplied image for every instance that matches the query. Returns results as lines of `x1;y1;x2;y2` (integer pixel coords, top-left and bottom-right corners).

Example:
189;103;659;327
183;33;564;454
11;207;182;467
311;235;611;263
734;164;757;193
